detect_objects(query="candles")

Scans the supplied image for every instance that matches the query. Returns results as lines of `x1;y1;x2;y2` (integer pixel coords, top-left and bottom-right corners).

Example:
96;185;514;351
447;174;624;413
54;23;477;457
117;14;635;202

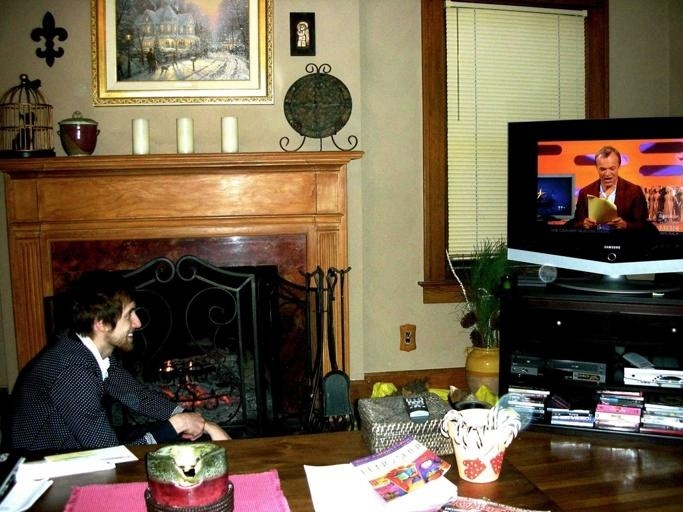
218;115;239;154
130;117;151;157
173;116;195;155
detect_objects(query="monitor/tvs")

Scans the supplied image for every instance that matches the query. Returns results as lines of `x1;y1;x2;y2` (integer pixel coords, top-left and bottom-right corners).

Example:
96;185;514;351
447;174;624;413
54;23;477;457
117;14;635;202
506;115;683;296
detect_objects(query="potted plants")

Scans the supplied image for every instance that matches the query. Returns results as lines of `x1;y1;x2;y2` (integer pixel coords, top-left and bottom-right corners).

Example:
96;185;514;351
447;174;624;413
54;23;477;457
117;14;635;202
459;235;516;404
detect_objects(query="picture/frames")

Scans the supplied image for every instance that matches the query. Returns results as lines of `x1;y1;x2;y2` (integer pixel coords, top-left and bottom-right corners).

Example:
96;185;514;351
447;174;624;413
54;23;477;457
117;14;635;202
86;1;275;108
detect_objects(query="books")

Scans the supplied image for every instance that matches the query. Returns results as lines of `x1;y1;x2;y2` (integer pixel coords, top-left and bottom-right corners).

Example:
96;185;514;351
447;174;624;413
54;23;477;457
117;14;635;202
305;435;457;512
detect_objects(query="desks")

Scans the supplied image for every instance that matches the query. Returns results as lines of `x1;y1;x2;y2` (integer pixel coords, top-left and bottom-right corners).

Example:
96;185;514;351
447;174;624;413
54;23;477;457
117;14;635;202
0;427;569;512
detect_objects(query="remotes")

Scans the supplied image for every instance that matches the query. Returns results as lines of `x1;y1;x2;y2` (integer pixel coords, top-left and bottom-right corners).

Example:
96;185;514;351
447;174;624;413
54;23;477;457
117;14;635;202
404;395;430;422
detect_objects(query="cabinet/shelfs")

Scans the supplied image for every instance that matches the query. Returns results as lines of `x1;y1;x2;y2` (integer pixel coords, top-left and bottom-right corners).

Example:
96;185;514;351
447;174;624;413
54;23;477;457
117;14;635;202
490;269;682;446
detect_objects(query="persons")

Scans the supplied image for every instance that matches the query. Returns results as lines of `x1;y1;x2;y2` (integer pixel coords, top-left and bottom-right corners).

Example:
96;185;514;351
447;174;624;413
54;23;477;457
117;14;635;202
7;269;232;448
574;146;647;229
